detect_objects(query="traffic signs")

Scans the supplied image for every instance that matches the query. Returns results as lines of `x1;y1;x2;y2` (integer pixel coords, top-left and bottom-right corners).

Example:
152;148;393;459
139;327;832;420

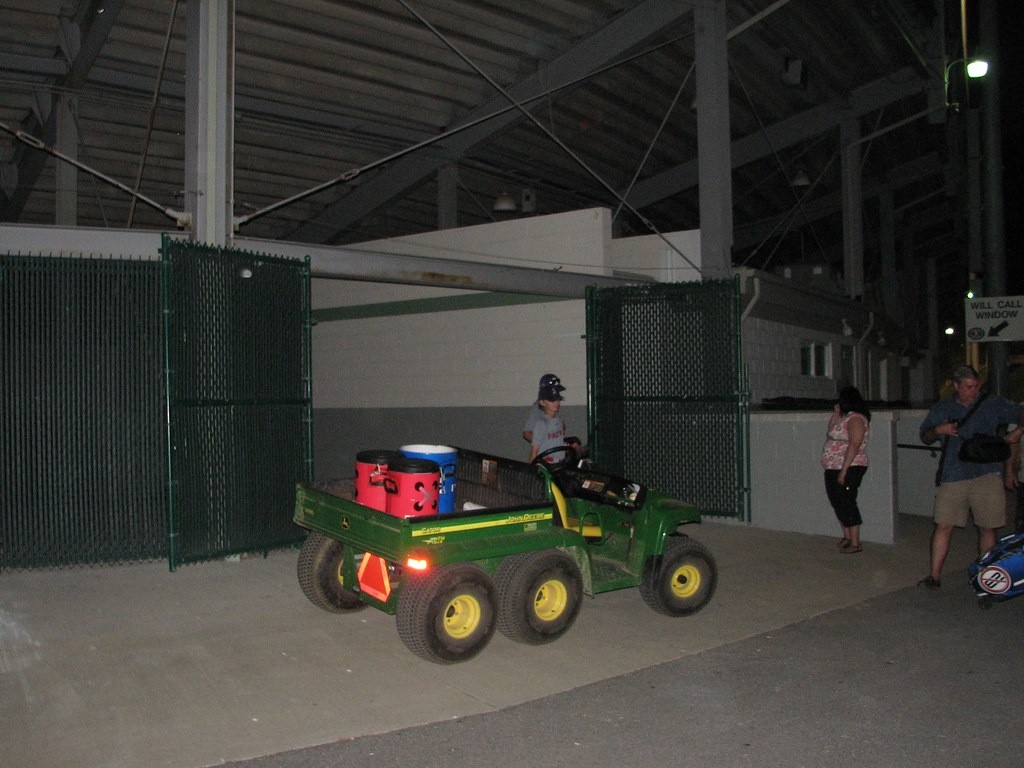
966;295;1024;342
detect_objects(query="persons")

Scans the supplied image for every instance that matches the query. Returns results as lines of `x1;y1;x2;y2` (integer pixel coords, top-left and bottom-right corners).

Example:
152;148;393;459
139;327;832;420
518;374;570;492
819;384;872;554
915;365;1024;587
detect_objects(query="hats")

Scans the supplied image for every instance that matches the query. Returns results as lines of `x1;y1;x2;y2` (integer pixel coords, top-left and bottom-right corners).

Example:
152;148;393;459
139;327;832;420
538;374;566;401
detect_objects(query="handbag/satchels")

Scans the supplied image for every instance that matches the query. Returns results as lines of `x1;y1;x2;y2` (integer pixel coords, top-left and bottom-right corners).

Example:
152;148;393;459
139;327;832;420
958;433;1012;464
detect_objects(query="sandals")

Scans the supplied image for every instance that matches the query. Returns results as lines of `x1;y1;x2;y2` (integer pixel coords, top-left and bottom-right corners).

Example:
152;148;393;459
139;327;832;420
837;536;862;553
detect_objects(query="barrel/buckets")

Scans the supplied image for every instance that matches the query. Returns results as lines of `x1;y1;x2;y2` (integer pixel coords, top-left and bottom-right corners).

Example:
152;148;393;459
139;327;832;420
397;444;458;514
354;448;406;514
385;457;440;520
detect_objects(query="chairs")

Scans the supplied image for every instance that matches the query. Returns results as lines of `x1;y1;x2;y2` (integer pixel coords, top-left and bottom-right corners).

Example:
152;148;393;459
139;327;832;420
547;480;607;546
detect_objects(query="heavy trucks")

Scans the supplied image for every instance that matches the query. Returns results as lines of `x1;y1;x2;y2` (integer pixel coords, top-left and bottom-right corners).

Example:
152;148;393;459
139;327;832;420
291;440;720;668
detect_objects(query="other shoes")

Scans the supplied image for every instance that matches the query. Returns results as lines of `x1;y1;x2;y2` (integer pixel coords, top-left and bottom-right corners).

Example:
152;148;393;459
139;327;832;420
917;576;941;589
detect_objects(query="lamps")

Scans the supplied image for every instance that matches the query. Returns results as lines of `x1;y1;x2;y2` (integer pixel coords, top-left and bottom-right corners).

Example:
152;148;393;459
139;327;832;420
793;170;811;187
841;318;853;337
493;191;518;212
878;332;886;346
942;45;992;112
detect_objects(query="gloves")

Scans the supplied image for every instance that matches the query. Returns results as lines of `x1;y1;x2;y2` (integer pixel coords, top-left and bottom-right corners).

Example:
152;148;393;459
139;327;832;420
563;437;582;446
